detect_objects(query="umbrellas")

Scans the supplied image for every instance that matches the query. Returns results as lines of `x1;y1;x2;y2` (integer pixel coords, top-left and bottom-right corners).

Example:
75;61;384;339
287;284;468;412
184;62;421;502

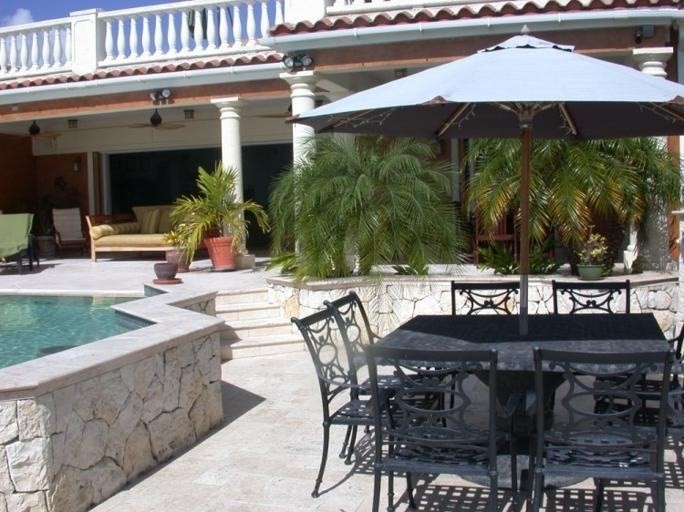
282;23;683;337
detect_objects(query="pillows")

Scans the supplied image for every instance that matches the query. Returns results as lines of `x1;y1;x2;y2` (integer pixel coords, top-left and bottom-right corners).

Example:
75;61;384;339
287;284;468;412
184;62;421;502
91;220;141;239
140;209;162;235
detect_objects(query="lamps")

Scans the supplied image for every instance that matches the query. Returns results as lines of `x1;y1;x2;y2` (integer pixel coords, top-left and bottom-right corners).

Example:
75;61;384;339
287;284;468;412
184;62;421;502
282;52;314;72
149;86;173;101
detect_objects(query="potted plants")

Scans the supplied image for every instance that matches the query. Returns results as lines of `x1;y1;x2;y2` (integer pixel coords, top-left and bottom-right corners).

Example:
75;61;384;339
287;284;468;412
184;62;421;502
166;160;269;272
162;229;191;272
573;226;609;280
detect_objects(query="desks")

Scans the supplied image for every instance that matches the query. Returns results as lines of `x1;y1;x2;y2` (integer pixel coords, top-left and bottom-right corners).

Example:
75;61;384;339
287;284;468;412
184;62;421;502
364;314;670;512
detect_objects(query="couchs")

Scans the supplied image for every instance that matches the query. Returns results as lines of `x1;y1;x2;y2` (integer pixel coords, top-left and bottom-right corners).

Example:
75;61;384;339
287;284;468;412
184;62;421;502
86;206;221;261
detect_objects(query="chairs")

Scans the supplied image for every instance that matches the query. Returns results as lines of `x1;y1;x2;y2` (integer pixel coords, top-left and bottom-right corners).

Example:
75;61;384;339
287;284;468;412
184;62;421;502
360;345;498;512
471;202;519;269
531;345;673;512
449;281;528;413
51;205;87;259
552;279;630;415
593;320;684;479
288;310;416;511
0;212;38;272
324;291;443;466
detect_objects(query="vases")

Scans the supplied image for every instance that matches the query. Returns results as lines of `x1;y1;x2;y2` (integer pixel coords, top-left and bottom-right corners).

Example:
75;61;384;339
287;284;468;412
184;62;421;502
153;262;182;284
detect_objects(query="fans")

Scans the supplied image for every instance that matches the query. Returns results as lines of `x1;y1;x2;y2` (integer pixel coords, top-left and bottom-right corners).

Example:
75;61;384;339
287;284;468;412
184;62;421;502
127;107;185;131
11;119;65;143
